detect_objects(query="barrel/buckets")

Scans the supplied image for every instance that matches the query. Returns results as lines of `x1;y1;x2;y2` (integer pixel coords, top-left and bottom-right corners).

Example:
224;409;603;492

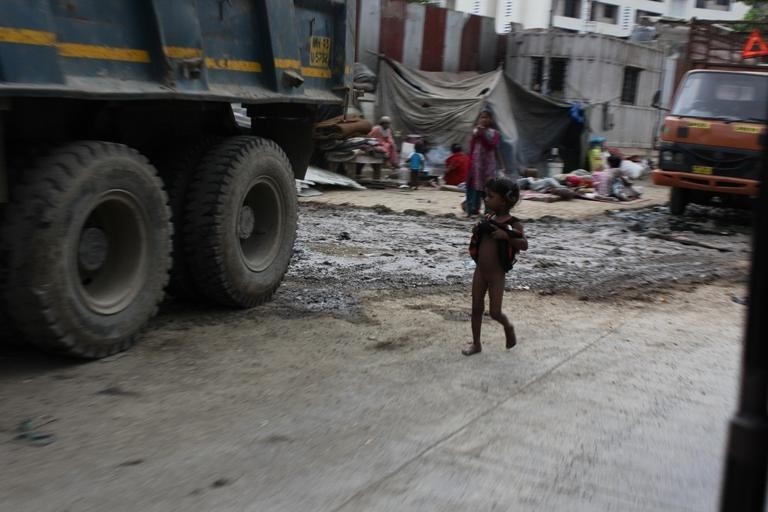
548;162;564;177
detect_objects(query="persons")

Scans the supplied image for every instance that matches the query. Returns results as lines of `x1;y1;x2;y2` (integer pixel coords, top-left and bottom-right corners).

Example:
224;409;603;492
461;177;528;356
460;109;499;218
442;143;472;186
597;154;633;201
405;142;425;190
517;168;600;200
367;115;397;168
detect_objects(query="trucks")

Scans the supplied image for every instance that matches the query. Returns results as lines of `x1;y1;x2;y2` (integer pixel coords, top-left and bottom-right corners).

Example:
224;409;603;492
651;16;768;216
0;0;357;358
